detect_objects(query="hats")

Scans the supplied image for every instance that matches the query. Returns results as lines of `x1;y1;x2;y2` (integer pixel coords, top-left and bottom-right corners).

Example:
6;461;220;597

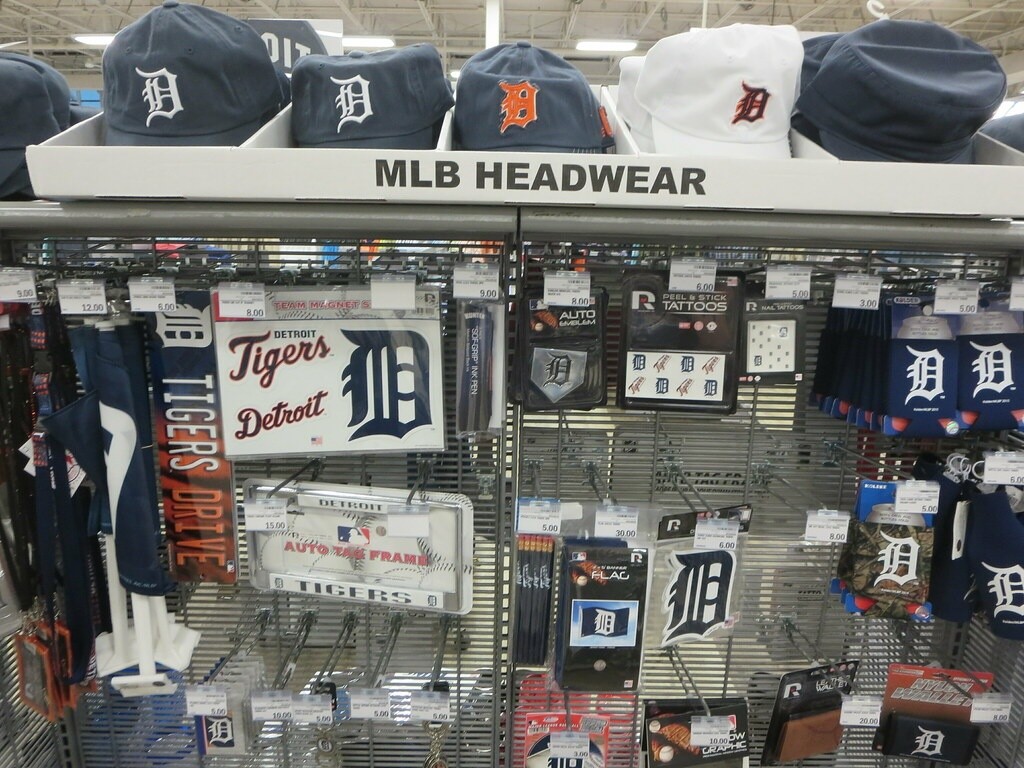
452;42;616;154
102;0;291;146
791;19;1007;164
70;105;102;125
291;43;455;149
0;53;69;201
633;22;805;159
616;56;656;153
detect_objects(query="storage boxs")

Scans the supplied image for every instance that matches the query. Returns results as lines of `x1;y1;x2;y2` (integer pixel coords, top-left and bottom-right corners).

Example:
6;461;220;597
22;86;1024;220
